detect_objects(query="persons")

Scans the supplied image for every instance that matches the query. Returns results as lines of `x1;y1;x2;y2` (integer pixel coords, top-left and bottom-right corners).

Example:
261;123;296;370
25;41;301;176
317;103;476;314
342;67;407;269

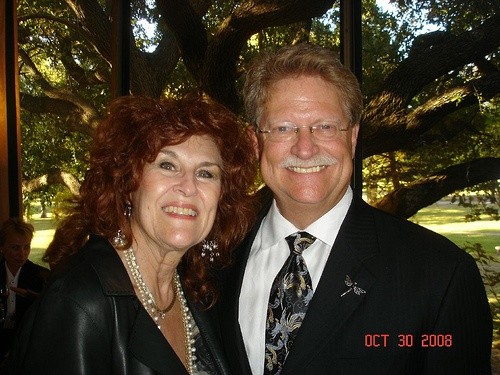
0;218;55;358
0;92;258;375
198;43;493;375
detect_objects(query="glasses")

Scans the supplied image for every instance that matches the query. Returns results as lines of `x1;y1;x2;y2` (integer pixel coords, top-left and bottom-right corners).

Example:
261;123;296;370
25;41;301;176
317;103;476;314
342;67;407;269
254;123;350;141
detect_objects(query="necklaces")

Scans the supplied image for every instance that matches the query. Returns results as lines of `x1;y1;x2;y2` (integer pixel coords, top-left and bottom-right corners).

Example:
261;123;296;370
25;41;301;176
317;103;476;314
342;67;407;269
119;233;197;375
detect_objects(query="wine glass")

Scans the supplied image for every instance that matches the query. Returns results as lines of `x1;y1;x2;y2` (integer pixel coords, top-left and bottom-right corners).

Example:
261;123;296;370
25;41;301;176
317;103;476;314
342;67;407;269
0;283;9;321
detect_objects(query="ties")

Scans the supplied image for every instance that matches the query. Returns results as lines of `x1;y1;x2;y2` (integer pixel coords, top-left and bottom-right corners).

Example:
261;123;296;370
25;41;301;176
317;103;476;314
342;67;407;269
264;232;317;375
